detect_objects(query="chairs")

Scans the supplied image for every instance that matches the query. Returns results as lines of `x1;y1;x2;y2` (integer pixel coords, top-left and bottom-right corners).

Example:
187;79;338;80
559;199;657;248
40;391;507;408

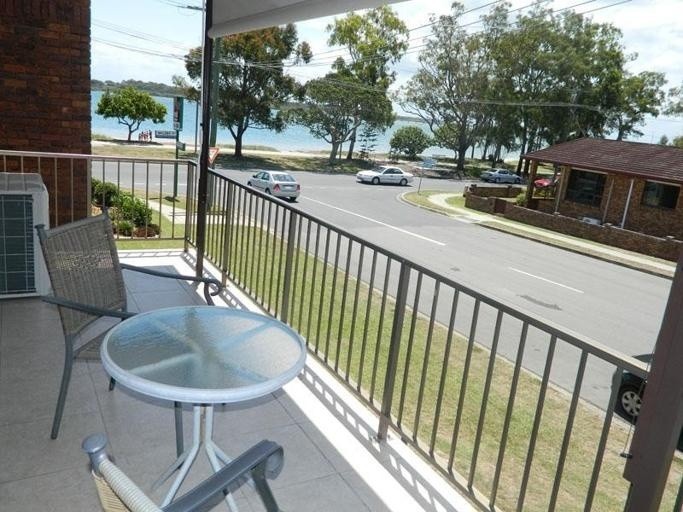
26;201;225;469
73;436;283;511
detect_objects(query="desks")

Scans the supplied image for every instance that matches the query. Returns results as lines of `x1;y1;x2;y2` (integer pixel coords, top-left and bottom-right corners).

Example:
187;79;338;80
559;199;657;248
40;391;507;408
97;305;305;511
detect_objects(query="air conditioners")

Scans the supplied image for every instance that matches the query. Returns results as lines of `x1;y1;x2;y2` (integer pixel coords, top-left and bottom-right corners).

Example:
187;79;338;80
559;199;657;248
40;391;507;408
582;215;600;225
0;171;51;302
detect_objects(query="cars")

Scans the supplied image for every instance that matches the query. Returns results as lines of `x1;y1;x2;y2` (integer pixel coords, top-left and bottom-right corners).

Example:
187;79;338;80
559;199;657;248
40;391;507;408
610;347;682;451
245;169;301;202
478;166;522;185
355;165;414;186
533;172;562;196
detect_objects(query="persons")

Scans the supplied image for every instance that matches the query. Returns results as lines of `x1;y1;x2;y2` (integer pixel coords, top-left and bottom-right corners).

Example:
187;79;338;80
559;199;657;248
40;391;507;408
136;130;151;140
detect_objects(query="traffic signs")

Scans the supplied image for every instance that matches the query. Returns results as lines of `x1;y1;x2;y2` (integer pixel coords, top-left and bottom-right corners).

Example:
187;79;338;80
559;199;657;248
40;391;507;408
176;141;185;151
154;129;177;139
